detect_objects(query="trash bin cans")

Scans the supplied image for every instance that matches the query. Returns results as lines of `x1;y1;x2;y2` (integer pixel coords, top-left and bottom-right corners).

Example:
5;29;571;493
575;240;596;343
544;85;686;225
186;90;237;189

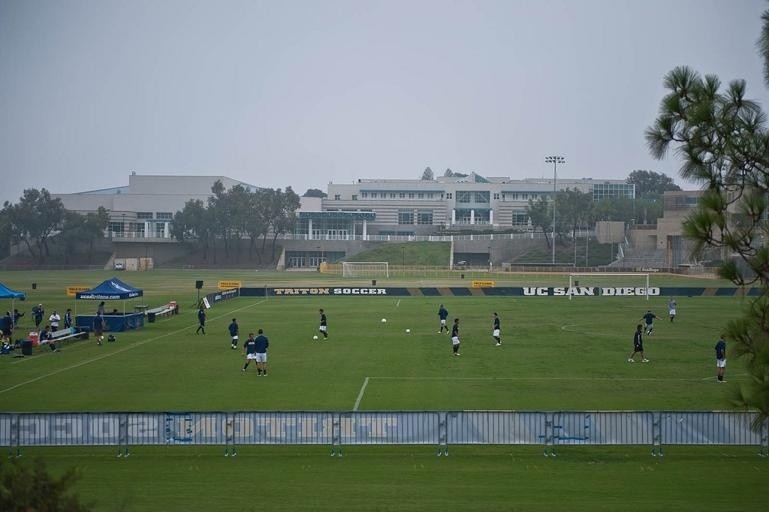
21;340;33;355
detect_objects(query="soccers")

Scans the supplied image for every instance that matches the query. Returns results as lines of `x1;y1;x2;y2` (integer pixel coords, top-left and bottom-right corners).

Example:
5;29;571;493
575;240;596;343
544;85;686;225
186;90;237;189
313;336;318;341
647;330;652;334
382;318;386;323
405;329;410;333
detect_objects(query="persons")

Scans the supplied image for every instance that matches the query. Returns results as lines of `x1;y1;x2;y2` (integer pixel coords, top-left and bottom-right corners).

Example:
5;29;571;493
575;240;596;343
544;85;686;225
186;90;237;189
640;310;663;335
628;324;649;363
452;319;463;355
228;318;239;350
0;301;118;352
196;306;208;336
437;304;450;335
241;333;257;371
492;313;502;346
668;297;678;322
319;309;328;340
254;329;269;376
715;335;728;382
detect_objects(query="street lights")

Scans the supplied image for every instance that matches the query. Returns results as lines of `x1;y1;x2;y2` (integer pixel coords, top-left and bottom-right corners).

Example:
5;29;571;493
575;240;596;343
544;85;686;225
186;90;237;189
400;245;406;271
121;213;126;235
316;246;320;267
488;247;492;272
542;155;567;263
145;245;149;268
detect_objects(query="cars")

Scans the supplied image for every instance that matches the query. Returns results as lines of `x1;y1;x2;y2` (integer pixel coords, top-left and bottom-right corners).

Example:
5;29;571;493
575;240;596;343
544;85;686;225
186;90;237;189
114;263;124;269
455;259;470;270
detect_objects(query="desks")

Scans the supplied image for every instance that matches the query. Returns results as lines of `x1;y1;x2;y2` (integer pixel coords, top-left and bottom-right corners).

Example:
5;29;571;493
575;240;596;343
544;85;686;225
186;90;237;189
78;312;145;331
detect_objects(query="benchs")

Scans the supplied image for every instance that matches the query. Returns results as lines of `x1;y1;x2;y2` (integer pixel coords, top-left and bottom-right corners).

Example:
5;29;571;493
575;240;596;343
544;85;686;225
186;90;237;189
39;327;86;349
147;301;179;324
